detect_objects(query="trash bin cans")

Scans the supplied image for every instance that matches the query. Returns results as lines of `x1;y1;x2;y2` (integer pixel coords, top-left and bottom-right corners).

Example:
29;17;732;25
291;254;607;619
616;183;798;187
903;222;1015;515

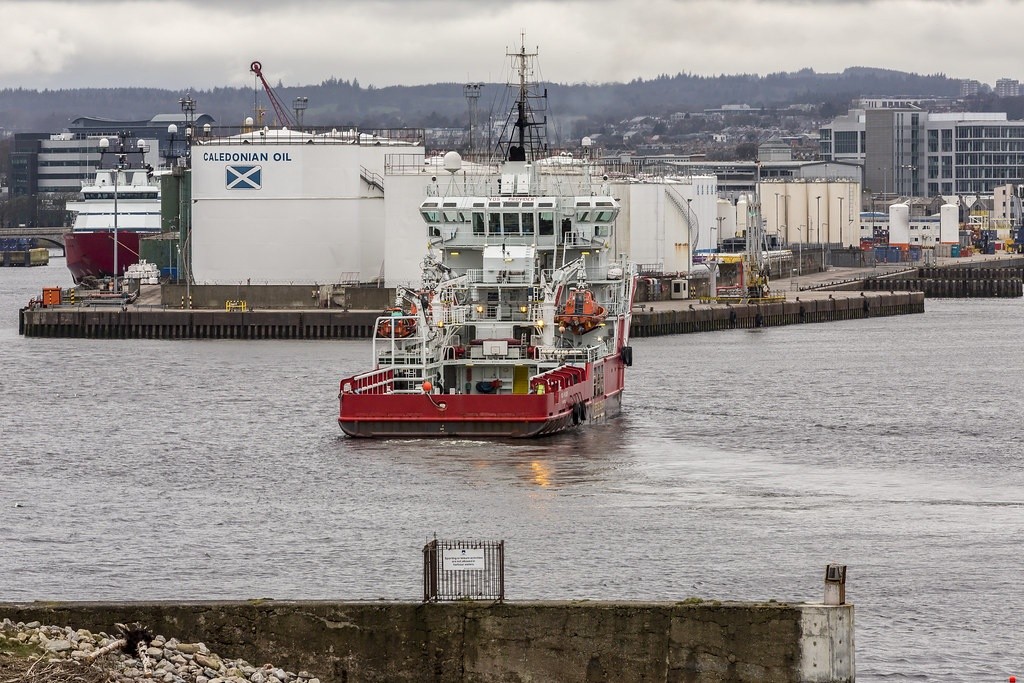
127;277;142;297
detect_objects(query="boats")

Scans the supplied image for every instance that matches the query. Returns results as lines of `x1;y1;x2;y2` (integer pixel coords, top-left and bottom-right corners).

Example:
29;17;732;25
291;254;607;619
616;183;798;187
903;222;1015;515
337;32;638;438
63;124;212;285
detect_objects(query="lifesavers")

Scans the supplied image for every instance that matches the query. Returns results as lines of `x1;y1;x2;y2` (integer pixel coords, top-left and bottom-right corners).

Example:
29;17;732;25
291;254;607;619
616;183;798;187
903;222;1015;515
756;314;762;323
573;403;581;423
802;307;805;316
730;310;736;322
628;346;632;365
864;301;869;311
622;346;630;364
580;403;587;419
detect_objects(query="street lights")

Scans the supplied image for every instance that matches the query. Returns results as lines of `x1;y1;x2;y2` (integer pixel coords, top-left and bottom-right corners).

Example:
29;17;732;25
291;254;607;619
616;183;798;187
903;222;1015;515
878;167;893;222
775;193;780;249
822;223;828;270
710;227;716;252
754;160;761;203
816;196;821;244
716;216;725;253
797;224;806;276
902;165;916;222
838;197;844;248
777;224;786;278
871;197;876;238
688;199;692;300
